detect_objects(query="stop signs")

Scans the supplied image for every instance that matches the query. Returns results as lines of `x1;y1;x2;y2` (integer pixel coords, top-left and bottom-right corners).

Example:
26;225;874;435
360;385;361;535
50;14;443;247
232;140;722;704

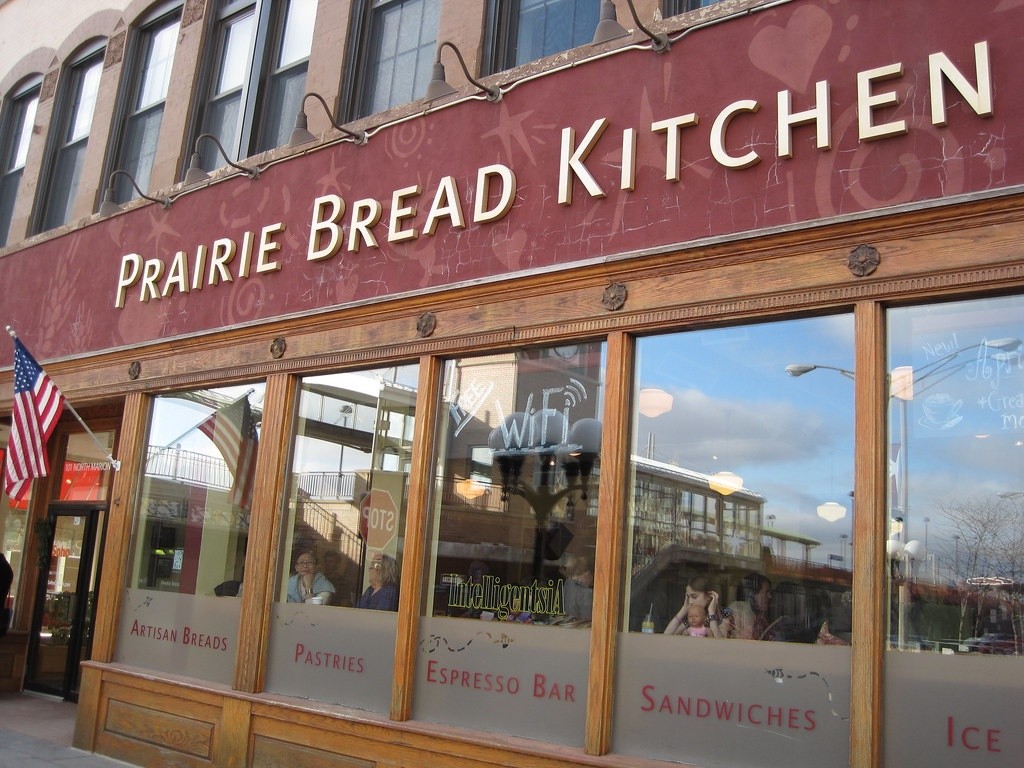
358;489;397;551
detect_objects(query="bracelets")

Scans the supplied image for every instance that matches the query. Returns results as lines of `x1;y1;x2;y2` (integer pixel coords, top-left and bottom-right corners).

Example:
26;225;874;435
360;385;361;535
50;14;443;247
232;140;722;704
708;615;718;621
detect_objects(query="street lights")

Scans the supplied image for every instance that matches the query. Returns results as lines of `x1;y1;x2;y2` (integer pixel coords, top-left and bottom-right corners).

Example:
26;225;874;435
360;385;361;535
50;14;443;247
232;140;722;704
849;491;855;567
786;338;1023;652
922;517;931;580
335;404;353;500
841;533;848;571
896;516;904;572
489;409;601;625
951;534;961;582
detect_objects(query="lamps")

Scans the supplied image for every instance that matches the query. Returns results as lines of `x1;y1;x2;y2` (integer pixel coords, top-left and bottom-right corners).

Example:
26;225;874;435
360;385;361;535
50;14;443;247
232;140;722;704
422;40;500;104
97;169;173;219
457;478;485;500
590;0;669;51
709;470;744;495
817;500;845;522
287;92;365;148
891;517;903;536
638;386;673;417
183;134;261;186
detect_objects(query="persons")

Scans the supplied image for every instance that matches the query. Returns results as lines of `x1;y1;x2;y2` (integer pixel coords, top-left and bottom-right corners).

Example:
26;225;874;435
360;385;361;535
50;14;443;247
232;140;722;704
562;547;595;622
0;552;14;637
974;579;1023;656
729;574;775;640
287;550;337;605
357;552;400;612
663;575;733;638
681;604;714;637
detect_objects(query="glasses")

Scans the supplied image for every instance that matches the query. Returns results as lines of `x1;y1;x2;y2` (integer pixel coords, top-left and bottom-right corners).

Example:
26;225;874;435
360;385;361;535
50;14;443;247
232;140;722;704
296;560;315;567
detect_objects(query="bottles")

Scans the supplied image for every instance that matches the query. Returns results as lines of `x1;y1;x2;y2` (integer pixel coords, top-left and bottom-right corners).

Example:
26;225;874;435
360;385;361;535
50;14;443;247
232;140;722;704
642;615;654;634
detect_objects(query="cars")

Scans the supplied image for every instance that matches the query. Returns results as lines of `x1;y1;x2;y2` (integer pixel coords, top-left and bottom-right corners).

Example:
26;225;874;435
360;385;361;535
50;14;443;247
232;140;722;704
963;631;1023;653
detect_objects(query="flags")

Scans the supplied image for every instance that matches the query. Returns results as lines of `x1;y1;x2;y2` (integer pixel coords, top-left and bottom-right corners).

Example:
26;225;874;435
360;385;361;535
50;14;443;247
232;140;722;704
3;336;67;502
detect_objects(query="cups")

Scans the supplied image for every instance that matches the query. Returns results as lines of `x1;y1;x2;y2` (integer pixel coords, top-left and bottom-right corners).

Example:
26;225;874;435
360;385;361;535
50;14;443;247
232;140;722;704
311;597;323;604
